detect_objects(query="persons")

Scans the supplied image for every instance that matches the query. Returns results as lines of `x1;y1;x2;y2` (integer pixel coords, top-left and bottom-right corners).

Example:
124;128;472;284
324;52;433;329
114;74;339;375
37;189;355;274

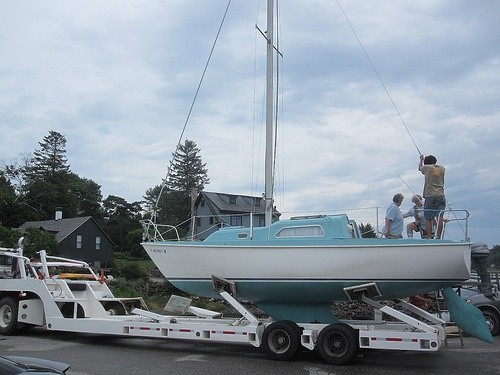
13;257;40;279
409;293;432;321
418;155;445;240
382;194;404;239
403;194;437;239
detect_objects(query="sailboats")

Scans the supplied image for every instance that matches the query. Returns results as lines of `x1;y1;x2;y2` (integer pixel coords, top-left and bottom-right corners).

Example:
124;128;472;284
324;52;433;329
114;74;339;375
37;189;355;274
139;0;494;344
